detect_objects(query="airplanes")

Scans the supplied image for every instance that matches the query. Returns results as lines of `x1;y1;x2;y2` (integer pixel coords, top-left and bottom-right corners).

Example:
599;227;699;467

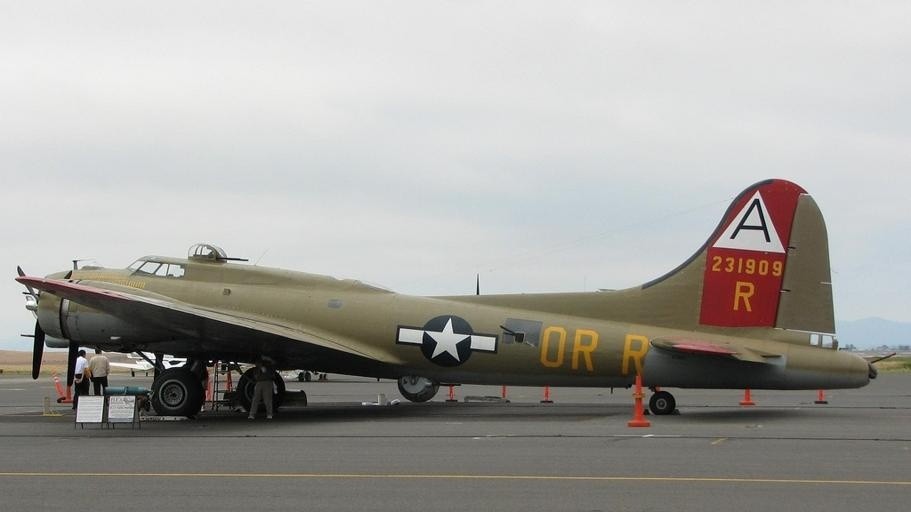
14;178;896;417
104;350;240;378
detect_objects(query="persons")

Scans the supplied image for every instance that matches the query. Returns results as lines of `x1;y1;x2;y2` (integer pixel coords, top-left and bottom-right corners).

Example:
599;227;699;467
248;356;276;420
89;349;110;396
73;350;90;408
